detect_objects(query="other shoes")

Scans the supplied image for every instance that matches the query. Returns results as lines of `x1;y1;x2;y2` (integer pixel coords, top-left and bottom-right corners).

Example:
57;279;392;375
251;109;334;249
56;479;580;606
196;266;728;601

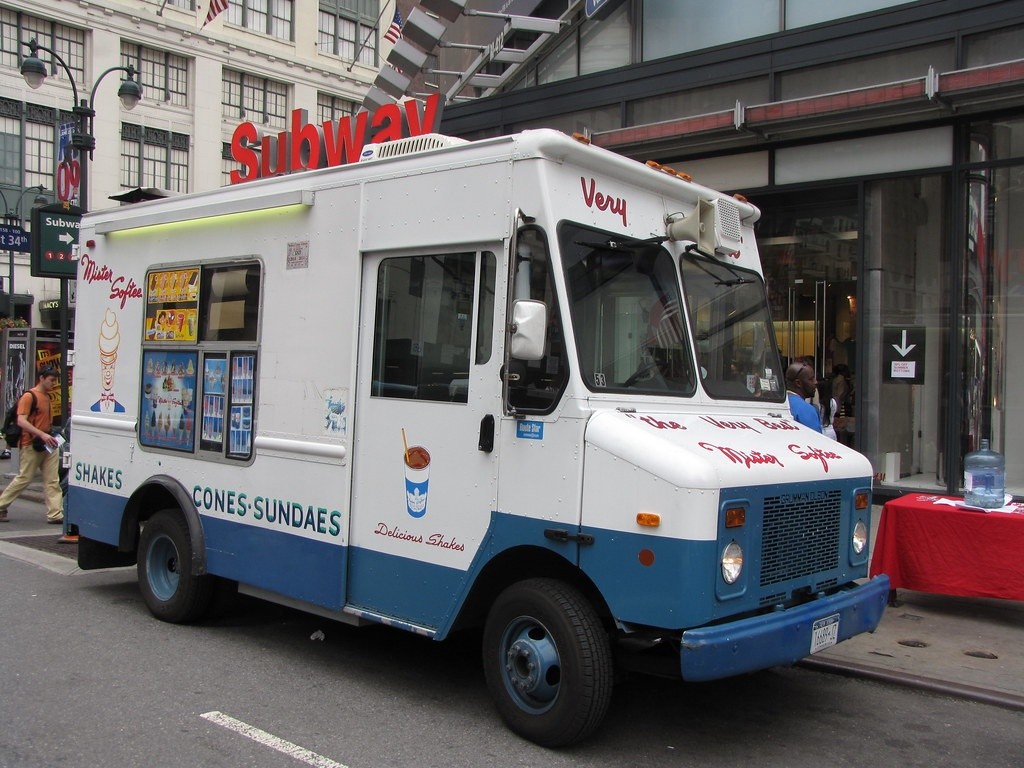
48;518;63;524
0;511;9;522
0;450;11;458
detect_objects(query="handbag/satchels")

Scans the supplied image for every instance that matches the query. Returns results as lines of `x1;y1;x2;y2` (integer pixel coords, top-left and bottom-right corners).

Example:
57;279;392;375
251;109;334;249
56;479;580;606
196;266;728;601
33;432;52;452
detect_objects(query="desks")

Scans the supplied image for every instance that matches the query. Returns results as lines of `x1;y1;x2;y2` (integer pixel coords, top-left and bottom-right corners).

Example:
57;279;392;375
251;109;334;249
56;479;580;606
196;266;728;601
870;492;1024;606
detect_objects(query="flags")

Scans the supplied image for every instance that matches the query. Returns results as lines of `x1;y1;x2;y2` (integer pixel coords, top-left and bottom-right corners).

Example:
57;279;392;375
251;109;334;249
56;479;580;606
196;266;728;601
200;0;229;30
645;277;684;351
384;5;403;44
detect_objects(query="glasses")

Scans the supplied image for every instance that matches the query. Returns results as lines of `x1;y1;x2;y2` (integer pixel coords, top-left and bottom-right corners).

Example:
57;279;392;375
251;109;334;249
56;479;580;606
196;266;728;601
43;366;53;377
793;361;809;382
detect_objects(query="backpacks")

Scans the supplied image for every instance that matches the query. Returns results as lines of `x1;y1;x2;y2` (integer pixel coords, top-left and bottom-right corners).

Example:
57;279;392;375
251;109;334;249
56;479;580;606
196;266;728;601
4;390;52;447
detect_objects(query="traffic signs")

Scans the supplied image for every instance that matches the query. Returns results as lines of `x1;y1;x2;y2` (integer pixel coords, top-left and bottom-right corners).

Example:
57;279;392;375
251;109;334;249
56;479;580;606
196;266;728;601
30;201;89;280
880;323;926;386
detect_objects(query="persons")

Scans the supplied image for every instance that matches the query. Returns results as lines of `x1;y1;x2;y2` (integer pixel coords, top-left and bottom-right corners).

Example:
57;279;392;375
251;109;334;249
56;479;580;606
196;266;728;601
733;347;854;449
0;366;78;544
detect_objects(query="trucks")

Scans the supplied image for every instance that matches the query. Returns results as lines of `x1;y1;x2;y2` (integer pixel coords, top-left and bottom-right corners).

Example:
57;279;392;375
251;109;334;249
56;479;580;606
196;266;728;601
60;132;889;749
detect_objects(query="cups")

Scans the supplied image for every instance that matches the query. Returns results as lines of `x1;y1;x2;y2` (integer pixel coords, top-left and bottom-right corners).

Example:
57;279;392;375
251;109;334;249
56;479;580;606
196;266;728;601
403;445;432;518
188;317;195;336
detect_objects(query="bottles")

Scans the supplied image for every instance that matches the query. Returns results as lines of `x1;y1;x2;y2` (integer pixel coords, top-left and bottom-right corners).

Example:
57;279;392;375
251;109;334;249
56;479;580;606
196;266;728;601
963;439;1005;509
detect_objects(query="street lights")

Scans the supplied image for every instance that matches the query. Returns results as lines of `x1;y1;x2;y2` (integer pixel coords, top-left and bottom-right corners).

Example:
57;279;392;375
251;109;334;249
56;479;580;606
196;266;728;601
0;183;47;322
17;38;142;214
